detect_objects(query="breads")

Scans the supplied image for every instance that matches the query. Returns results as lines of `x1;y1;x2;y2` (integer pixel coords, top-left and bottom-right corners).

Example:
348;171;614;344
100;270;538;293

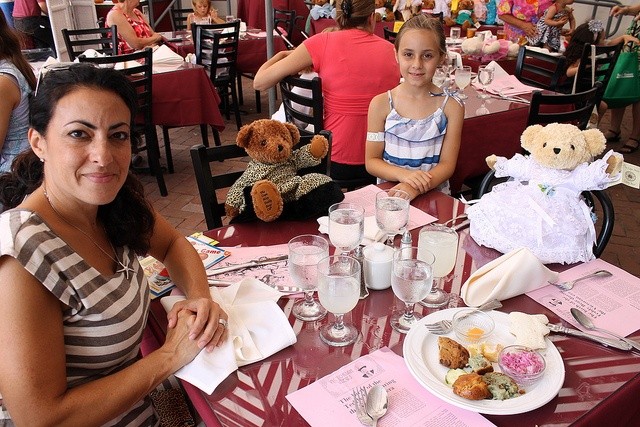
483;372;519;400
452;373;490;400
438;337;469;368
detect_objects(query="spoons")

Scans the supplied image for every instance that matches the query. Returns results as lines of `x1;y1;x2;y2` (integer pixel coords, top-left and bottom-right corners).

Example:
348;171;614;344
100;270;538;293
365;383;389;427
570;307;637;349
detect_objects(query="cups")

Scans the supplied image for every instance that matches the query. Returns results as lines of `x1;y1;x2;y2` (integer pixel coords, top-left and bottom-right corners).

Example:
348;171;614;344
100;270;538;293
362;242;396;289
452;309;496;344
498;345;547;386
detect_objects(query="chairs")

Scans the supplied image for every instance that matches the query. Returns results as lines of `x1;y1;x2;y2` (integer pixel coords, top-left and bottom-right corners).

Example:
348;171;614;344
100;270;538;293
276;71;325;139
188;129;336;234
522;81;605;165
169;5;195;30
272;8;295;43
569;37;626;105
59;25;118;61
380;26;402;47
72;45;169;198
160;24;225;174
472;158;615;265
510;43;574;92
187;18;254;130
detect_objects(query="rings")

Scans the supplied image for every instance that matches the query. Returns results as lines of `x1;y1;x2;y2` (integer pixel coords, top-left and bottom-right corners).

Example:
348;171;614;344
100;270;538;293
219;319;228;327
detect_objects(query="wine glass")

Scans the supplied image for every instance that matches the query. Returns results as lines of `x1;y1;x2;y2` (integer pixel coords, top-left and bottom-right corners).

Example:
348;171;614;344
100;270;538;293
442;55;457;80
478;65;495;99
317;255;361;345
455;66;471;98
390;246;435;335
450;28;461;48
329;202;365;257
416;224;459;307
376;190;410;244
287;234;329;321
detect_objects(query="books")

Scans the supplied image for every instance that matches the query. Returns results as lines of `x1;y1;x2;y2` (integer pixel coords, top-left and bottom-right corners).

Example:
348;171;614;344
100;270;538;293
139;233;231;300
471;60;544;99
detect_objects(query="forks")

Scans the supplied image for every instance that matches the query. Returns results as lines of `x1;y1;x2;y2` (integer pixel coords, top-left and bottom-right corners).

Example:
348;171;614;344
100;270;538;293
425;299;503;335
547;270;614;290
352;385;374;426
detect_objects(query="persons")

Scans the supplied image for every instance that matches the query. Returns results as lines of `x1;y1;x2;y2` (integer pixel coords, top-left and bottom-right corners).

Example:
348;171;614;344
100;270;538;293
0;7;37;213
13;0;57;56
187;0;226;30
604;3;640;153
365;13;465;203
104;0;162;56
0;1;37;62
271;27;341;130
0;64;228;427
525;0;576;52
253;0;401;193
555;19;640;125
497;0;552;43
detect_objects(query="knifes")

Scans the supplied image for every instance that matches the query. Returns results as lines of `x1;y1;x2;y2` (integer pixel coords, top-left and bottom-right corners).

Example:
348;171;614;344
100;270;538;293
203;254;289;273
206;278;304;294
546;323;634;354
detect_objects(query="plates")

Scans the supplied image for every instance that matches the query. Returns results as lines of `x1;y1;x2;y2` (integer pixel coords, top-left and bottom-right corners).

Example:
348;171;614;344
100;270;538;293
402;307;565;415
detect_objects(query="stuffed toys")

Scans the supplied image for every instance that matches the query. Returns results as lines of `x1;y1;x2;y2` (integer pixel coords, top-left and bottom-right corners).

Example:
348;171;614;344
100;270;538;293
392;0;424;21
459;123;624;265
225;119;333;222
375;0;395;23
444;0;477;30
447;3;459;19
305;0;336;35
462;33;520;62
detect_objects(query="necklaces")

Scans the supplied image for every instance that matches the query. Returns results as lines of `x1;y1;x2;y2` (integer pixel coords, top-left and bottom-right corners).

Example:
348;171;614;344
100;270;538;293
41;185;138;280
